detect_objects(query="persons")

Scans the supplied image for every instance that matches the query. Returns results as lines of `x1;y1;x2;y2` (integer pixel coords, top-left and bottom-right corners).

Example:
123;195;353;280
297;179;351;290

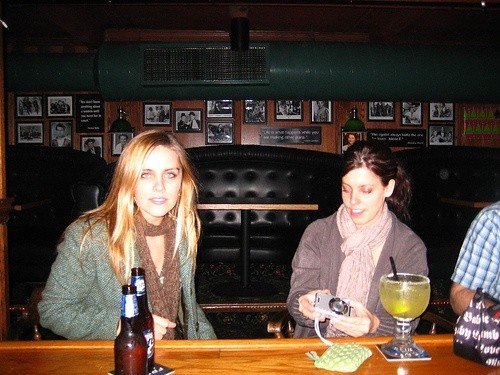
51;124;71;147
146;106;166;122
178;111;199;130
344;133;359;151
286;141;428;337
314;100;329;121
213;101;224;113
50;100;71;115
85;139;101;156
38;130;216;340
18;97;40;116
115;134;128;153
247;101;265;122
449;200;500;318
371;103;393;117
403;103;419;123
209;124;226;141
433;103;453;118
430;129;452;143
277;100;298;115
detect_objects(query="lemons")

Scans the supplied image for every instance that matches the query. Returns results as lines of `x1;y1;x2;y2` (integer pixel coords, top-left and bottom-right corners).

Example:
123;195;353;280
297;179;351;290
392;304;409;313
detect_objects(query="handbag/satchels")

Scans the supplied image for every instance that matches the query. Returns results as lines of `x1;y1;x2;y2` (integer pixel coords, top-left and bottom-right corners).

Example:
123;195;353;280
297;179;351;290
453;287;500;368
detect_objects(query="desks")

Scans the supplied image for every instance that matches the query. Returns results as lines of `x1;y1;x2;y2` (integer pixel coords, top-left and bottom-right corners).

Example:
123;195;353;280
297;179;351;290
9;197;53;304
440;196;496;211
196;199;320;297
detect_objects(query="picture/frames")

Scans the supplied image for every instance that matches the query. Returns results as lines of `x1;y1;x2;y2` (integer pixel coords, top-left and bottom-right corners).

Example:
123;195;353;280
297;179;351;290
243;100;268;124
311;101;333;123
368;101;455;146
15;94;104;158
205;119;235;145
341;126;363;155
143;101;173;125
111;132;134;156
174;107;204;132
275;100;304;120
205;99;235;118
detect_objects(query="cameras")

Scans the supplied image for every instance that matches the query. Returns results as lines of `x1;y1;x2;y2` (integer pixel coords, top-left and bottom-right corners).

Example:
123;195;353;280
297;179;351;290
315;293;351;319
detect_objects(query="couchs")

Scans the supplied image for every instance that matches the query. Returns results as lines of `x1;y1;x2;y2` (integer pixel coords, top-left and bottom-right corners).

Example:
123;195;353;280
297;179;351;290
6;144;500;279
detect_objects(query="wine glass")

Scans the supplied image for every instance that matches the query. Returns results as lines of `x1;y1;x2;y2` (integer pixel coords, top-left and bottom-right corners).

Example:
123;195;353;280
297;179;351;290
378;272;431;358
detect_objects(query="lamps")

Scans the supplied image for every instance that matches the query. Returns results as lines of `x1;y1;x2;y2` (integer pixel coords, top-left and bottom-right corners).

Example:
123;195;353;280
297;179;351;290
229;15;251;53
106;105;136;133
342;105;367;133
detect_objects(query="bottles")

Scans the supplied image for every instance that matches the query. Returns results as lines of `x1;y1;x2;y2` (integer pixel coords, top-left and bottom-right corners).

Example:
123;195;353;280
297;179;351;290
130;268;155;373
114;284;149;375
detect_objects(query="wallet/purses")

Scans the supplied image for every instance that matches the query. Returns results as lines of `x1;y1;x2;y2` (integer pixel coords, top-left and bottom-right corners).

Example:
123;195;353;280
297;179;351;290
305;342;372;372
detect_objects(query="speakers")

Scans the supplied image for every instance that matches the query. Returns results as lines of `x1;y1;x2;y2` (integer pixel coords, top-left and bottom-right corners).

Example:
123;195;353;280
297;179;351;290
230;17;250;51
